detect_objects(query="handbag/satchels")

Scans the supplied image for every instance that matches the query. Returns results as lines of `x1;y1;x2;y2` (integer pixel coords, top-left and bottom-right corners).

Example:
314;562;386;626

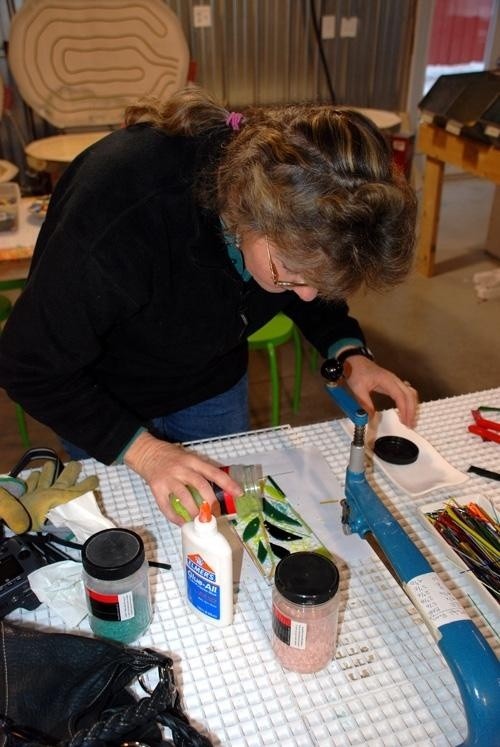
0;624;214;747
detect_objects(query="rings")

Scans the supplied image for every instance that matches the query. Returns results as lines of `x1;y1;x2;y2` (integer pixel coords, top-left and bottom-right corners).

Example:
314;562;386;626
402;380;413;388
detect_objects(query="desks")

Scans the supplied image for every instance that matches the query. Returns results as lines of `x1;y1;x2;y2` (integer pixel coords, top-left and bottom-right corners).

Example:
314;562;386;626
414;119;500;278
0;194;51;281
0;387;500;746
24;108;401;186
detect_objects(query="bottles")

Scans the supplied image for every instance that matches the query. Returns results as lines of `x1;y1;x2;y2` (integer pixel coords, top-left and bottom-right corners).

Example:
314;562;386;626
270;551;337;674
168;463;265;523
81;527;152;645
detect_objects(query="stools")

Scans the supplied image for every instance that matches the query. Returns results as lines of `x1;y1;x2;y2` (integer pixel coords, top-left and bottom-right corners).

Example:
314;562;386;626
247;313;302;426
309;343;316;374
0;294;29;448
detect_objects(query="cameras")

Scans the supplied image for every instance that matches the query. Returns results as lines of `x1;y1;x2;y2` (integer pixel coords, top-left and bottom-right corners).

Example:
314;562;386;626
0;531;49;620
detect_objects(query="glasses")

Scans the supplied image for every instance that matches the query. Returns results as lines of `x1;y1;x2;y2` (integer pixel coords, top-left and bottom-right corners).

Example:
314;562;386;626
266;236;309;291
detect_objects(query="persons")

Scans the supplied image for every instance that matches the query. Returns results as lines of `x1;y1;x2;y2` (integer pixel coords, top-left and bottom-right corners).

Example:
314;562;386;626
0;84;424;533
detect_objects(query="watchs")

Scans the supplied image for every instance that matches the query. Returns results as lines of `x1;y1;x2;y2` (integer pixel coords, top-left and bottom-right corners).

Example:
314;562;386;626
338;343;378;379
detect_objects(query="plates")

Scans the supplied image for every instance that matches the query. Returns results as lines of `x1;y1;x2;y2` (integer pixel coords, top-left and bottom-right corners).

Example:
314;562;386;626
416;492;500;614
339;407;469;499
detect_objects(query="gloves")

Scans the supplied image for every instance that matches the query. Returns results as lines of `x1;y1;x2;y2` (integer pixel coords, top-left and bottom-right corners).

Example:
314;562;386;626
0;460;99;536
